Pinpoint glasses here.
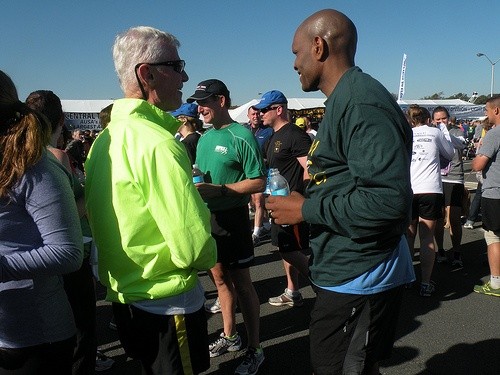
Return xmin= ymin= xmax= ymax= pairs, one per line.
xmin=260 ymin=106 xmax=278 ymax=113
xmin=135 ymin=59 xmax=187 ymax=101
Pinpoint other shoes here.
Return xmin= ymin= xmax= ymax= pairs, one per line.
xmin=464 ymin=223 xmax=474 ymax=229
xmin=251 ymin=221 xmax=273 ymax=248
xmin=109 ymin=320 xmax=117 ymax=330
xmin=436 ymin=247 xmax=464 ymax=261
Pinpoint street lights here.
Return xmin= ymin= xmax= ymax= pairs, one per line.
xmin=477 ymin=52 xmax=500 ymax=97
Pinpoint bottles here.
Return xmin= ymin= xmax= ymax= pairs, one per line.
xmin=268 ymin=168 xmax=291 ymax=227
xmin=191 ymin=164 xmax=206 ymax=184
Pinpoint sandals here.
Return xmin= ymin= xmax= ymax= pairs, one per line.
xmin=268 ymin=288 xmax=303 ymax=307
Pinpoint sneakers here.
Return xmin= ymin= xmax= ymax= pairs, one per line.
xmin=207 ymin=331 xmax=242 ymax=358
xmin=211 ymin=296 xmax=237 ymax=314
xmin=234 ymin=344 xmax=265 ymax=375
xmin=473 ymin=281 xmax=500 ymax=297
xmin=405 ymin=283 xmax=415 ymax=288
xmin=419 ymin=280 xmax=436 ymax=297
xmin=94 ymin=352 xmax=116 ymax=372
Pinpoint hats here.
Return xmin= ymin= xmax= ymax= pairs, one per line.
xmin=251 ymin=90 xmax=288 ymax=111
xmin=186 ymin=79 xmax=230 ymax=104
xmin=483 ymin=94 xmax=500 ymax=105
xmin=171 ymin=102 xmax=201 ymax=117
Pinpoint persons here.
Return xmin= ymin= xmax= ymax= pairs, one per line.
xmin=66 ymin=128 xmax=103 ymax=180
xmin=185 ymin=79 xmax=266 ymax=375
xmin=432 ymin=107 xmax=469 ymax=272
xmin=463 ymin=118 xmax=493 ymax=229
xmin=166 ymin=102 xmax=240 ymax=313
xmin=0 ymin=70 xmax=83 ymax=375
xmin=265 ymin=9 xmax=413 ymax=375
xmin=252 ymin=90 xmax=314 ymax=307
xmin=448 ymin=117 xmax=484 ymax=158
xmin=405 ymin=104 xmax=454 ymax=297
xmin=243 ymin=106 xmax=274 ymax=247
xmin=24 ymin=90 xmax=96 ymax=375
xmin=471 ymin=94 xmax=500 ymax=296
xmin=84 ymin=26 xmax=218 ymax=375
xmin=55 ymin=123 xmax=117 ymax=372
xmin=287 ymin=108 xmax=323 ymax=142
